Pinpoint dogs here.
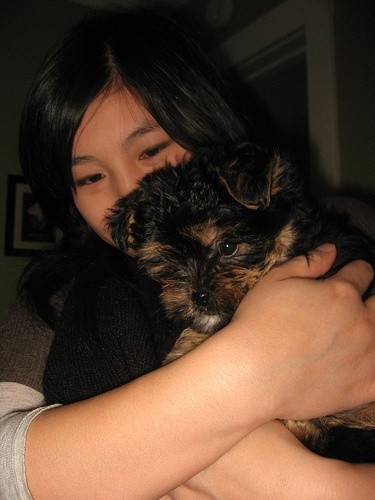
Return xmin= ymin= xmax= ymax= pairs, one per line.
xmin=101 ymin=140 xmax=373 ymax=464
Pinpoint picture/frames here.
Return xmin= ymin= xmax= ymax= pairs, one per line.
xmin=5 ymin=174 xmax=61 ymax=257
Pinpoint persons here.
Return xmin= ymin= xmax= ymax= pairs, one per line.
xmin=0 ymin=8 xmax=375 ymax=500
